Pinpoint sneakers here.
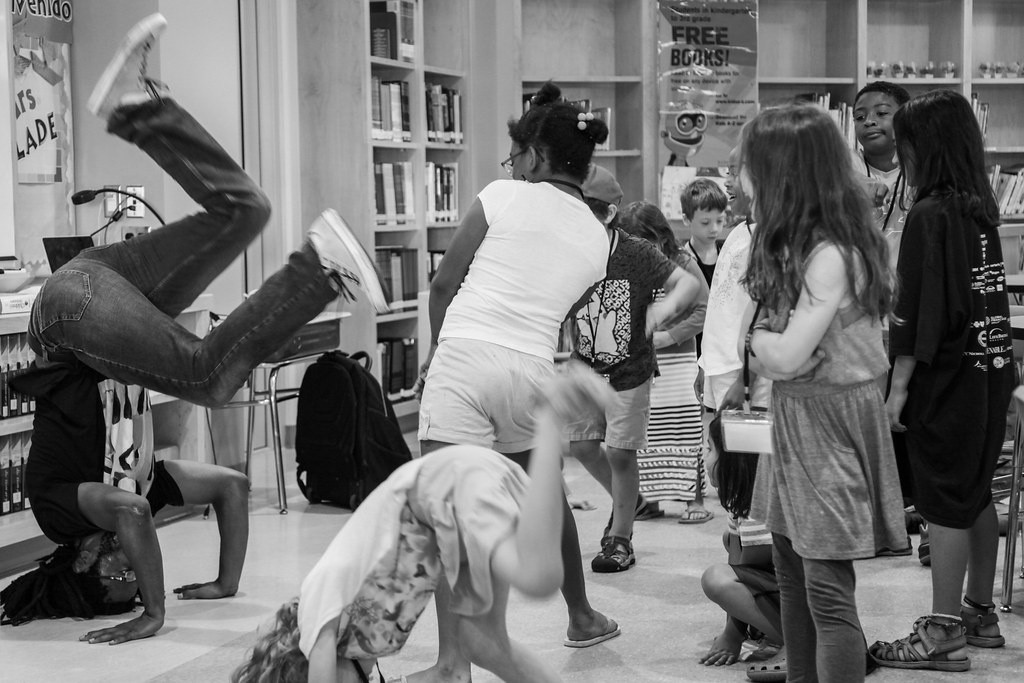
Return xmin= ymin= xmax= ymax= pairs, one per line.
xmin=309 ymin=207 xmax=391 ymax=314
xmin=87 ymin=12 xmax=167 ymax=122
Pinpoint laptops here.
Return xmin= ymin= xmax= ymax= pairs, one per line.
xmin=43 ymin=237 xmax=94 ymax=274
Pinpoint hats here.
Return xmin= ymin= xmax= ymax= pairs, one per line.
xmin=581 ymin=163 xmax=624 ymax=206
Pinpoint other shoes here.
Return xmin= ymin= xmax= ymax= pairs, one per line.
xmin=919 ymin=523 xmax=931 ymax=566
xmin=874 ymin=535 xmax=913 ymax=556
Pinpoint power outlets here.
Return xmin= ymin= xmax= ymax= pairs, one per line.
xmin=102 ymin=185 xmax=121 ymax=218
xmin=126 ymin=185 xmax=145 ymax=218
xmin=121 ymin=225 xmax=151 ymax=242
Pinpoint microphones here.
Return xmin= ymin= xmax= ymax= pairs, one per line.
xmin=71 ymin=189 xmax=166 ymax=244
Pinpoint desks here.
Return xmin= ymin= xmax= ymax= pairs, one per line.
xmin=1006 ymin=274 xmax=1024 ymax=305
xmin=1007 ymin=315 xmax=1024 ymax=339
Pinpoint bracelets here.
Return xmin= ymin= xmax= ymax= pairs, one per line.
xmin=746 ymin=327 xmax=771 ymax=357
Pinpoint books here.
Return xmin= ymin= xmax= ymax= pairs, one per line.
xmin=372 ymin=74 xmax=412 ymax=142
xmin=524 ymin=92 xmax=611 ymax=151
xmin=426 ymin=84 xmax=463 ymax=144
xmin=376 ymin=246 xmax=418 ymax=313
xmin=373 ymin=161 xmax=414 ymax=225
xmin=428 ymin=250 xmax=448 ymax=283
xmin=425 ymin=161 xmax=460 ymax=223
xmin=800 ymin=90 xmax=855 ymax=151
xmin=379 ymin=340 xmax=417 ymax=402
xmin=974 ymin=92 xmax=1024 ymax=216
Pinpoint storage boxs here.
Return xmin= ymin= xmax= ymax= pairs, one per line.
xmin=0 ymin=332 xmax=38 ymax=421
xmin=0 ymin=294 xmax=31 ymax=314
xmin=262 ymin=318 xmax=340 ymax=363
xmin=0 ymin=429 xmax=32 ymax=516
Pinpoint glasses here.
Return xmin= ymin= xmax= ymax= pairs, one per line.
xmin=503 ymin=145 xmax=544 ymax=177
xmin=98 ymin=569 xmax=137 ymax=583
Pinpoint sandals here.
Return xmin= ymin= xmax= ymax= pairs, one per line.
xmin=602 ymin=492 xmax=645 ymax=536
xmin=868 ymin=611 xmax=971 ymax=672
xmin=591 ymin=534 xmax=635 ymax=573
xmin=959 ymin=595 xmax=1006 ymax=646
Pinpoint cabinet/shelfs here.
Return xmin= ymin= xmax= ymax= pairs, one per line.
xmin=0 ymin=292 xmax=213 ymax=578
xmin=757 ymin=0 xmax=1024 ymax=221
xmin=295 ymin=0 xmax=471 ymax=443
xmin=496 ymin=0 xmax=656 ymax=208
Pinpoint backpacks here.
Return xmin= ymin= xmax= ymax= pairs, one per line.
xmin=295 ymin=350 xmax=413 ymax=512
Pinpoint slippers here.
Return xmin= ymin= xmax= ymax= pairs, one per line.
xmin=679 ymin=508 xmax=714 ymax=524
xmin=564 ymin=616 xmax=622 ymax=648
xmin=746 ymin=644 xmax=788 ymax=682
xmin=636 ymin=509 xmax=665 ymax=520
xmin=386 ymin=675 xmax=408 ymax=683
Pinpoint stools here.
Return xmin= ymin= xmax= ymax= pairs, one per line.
xmin=1001 ymin=384 xmax=1024 ymax=613
xmin=204 ymin=348 xmax=350 ymax=519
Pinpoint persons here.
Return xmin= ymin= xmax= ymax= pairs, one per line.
xmin=622 ymin=199 xmax=713 ymax=523
xmin=868 ymin=89 xmax=1006 ymax=672
xmin=739 ymin=107 xmax=909 ymax=683
xmin=702 ymin=129 xmax=786 ymax=680
xmin=569 ymin=163 xmax=698 ymax=570
xmin=232 ymin=360 xmax=628 ymax=683
xmin=385 ymin=82 xmax=610 ymax=683
xmin=678 ymin=180 xmax=727 ymax=283
xmin=1 ymin=14 xmax=392 ymax=646
xmin=853 ymin=82 xmax=931 ymax=562
